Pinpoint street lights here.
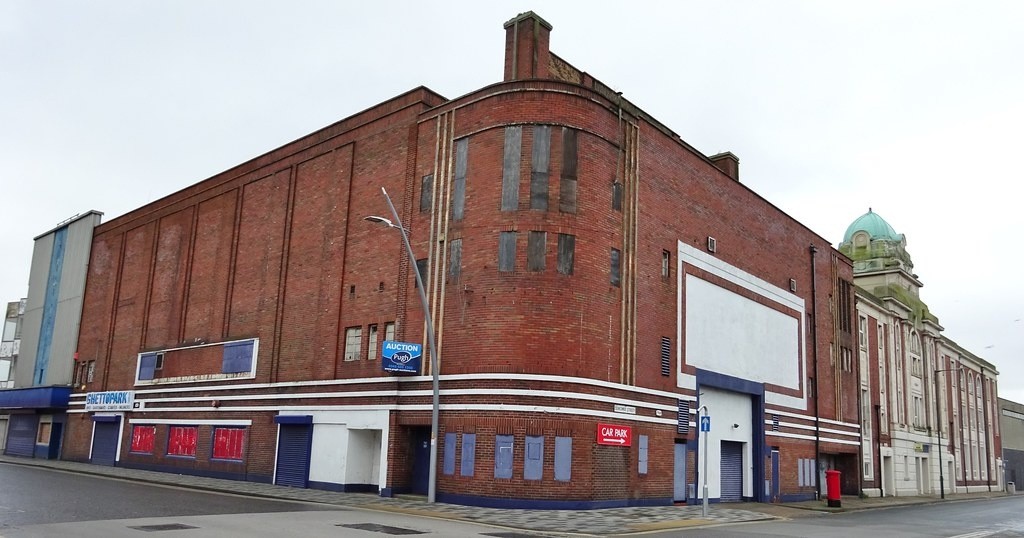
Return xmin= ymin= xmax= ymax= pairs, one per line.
xmin=363 ymin=187 xmax=440 ymax=504
xmin=934 ymin=367 xmax=962 ymax=498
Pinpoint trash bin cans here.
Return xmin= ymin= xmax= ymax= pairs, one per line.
xmin=1007 ymin=481 xmax=1015 ymax=495
